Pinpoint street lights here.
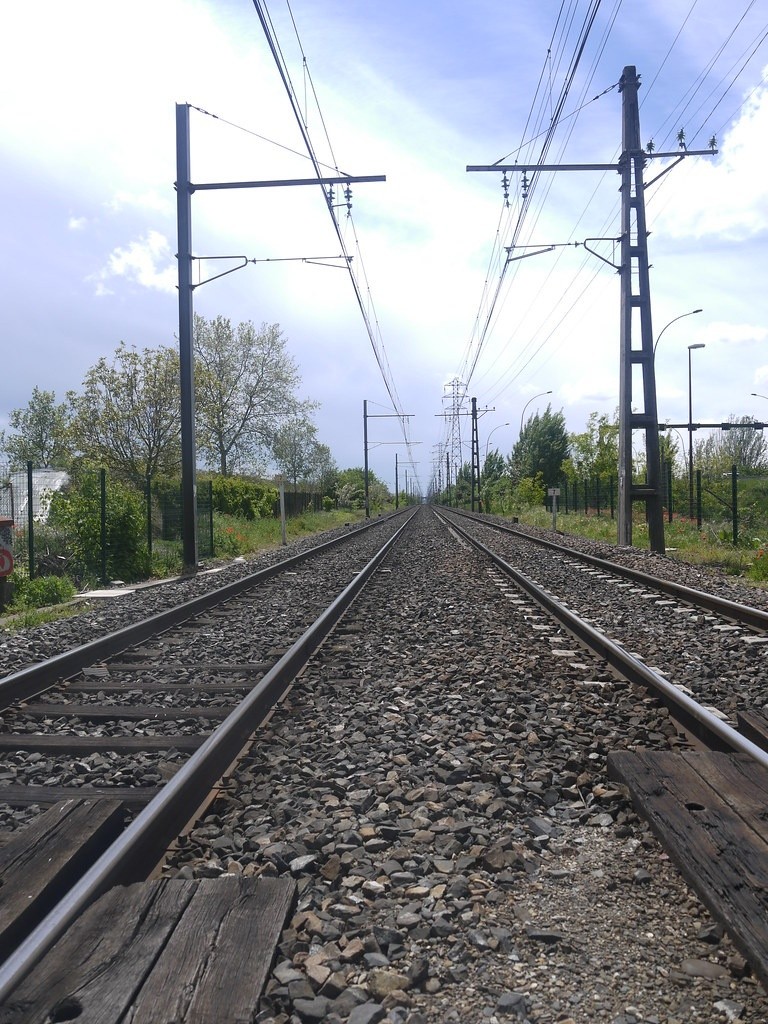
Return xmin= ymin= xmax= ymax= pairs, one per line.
xmin=521 ymin=390 xmax=553 ymax=439
xmin=687 ymin=342 xmax=707 ymax=520
xmin=486 ymin=423 xmax=510 ymax=457
